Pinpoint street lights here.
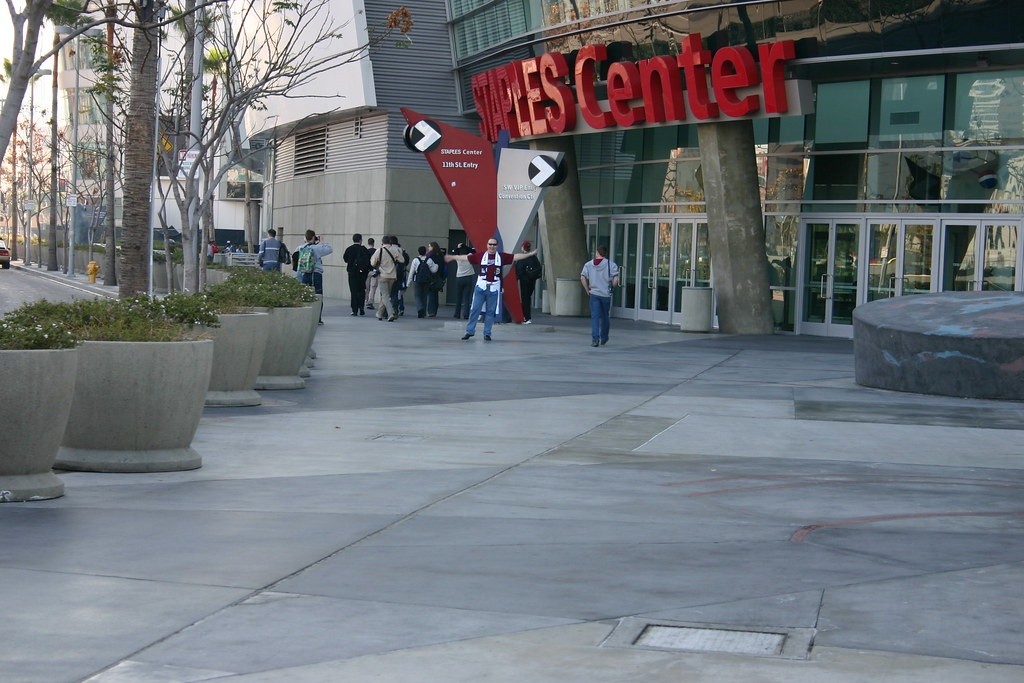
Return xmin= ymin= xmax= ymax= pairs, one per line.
xmin=24 ymin=69 xmax=53 ymax=266
xmin=52 ymin=24 xmax=102 ymax=277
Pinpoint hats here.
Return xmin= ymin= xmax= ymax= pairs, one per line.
xmin=521 ymin=241 xmax=531 ymax=249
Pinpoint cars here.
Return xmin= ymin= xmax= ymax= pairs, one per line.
xmin=0 ymin=240 xmax=11 ymax=269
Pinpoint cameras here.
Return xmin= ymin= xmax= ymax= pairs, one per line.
xmin=372 ymin=269 xmax=380 ymax=277
xmin=316 ymin=236 xmax=320 ymax=240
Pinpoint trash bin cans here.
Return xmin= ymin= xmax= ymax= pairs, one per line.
xmin=680 ymin=286 xmax=712 ymax=334
xmin=554 ymin=277 xmax=583 ymax=318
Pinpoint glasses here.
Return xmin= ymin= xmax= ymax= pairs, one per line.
xmin=442 ymin=252 xmax=445 ymax=253
xmin=488 ymin=243 xmax=497 ymax=246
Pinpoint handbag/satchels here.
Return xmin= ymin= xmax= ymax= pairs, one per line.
xmin=279 ymin=242 xmax=291 ymax=265
xmin=397 ymin=264 xmax=403 ymax=277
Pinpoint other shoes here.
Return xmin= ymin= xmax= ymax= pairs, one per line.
xmin=360 ymin=312 xmax=365 ymax=315
xmin=388 ymin=317 xmax=394 ymax=322
xmin=484 ymin=334 xmax=491 ymax=341
xmin=454 ymin=314 xmax=460 ymax=319
xmin=367 ymin=303 xmax=374 ymax=309
xmin=601 ymin=336 xmax=609 ymax=345
xmin=417 ymin=306 xmax=425 ymax=318
xmin=464 ymin=315 xmax=469 ymax=319
xmin=591 ymin=343 xmax=598 ymax=347
xmin=398 ymin=310 xmax=404 ymax=316
xmin=522 ymin=320 xmax=531 ymax=324
xmin=461 ymin=333 xmax=474 ymax=340
xmin=318 ymin=321 xmax=323 ymax=325
xmin=428 ymin=312 xmax=437 ymax=317
xmin=351 ymin=312 xmax=357 ymax=316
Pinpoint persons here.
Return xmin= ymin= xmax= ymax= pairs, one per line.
xmin=444 ymin=237 xmax=541 ymax=341
xmin=580 ymin=246 xmax=619 ymax=348
xmin=206 ymin=228 xmax=474 ymax=322
xmin=514 ymin=241 xmax=542 ymax=324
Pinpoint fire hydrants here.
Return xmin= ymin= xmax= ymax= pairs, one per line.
xmin=86 ymin=261 xmax=102 ymax=285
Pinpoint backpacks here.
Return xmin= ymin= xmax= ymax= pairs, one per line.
xmin=297 ymin=247 xmax=317 ymax=274
xmin=415 ymin=257 xmax=431 ymax=284
xmin=524 ymin=257 xmax=542 ymax=280
